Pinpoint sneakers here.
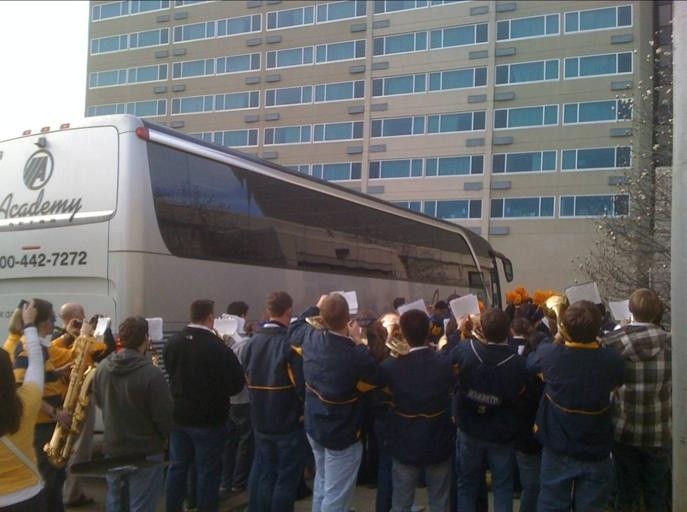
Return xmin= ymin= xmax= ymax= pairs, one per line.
xmin=410 ymin=503 xmax=425 ymax=512
xmin=220 ymin=484 xmax=245 ymax=492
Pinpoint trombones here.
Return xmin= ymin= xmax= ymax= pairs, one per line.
xmin=381 ymin=314 xmax=409 ymax=359
xmin=543 ymin=295 xmax=572 ymax=342
xmin=305 ymin=314 xmax=369 ymax=346
xmin=470 ymin=314 xmax=489 ymax=347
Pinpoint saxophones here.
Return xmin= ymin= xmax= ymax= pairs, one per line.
xmin=43 ymin=314 xmax=104 ymax=471
xmin=212 ymin=327 xmax=235 ymax=347
xmin=148 ymin=336 xmax=160 ymax=368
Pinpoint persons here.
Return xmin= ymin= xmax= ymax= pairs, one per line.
xmin=162 ymin=298 xmax=245 ymax=512
xmin=350 ymin=308 xmax=391 ymax=490
xmin=429 ymin=300 xmax=461 ymax=352
xmin=369 ymin=313 xmax=425 ymax=511
xmin=446 ymin=307 xmax=526 ymax=511
xmin=527 ymin=300 xmax=628 ymax=511
xmin=513 ymin=330 xmax=547 ymax=512
xmin=1 ymin=307 xmax=24 ymax=366
xmin=287 ymin=292 xmax=379 ymax=511
xmin=374 ymin=309 xmax=457 ymax=511
xmin=0 ymin=298 xmax=51 ymax=511
xmin=90 ymin=314 xmax=176 ymax=511
xmin=599 ymin=287 xmax=672 ymax=511
xmin=50 ymin=301 xmax=117 ymax=508
xmin=218 ymin=301 xmax=255 ymax=494
xmin=508 ymin=316 xmax=531 ymax=348
xmin=239 ymin=290 xmax=305 ymax=512
xmin=13 ymin=298 xmax=83 ymax=512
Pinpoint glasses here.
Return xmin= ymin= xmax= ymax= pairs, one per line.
xmin=45 ymin=314 xmax=57 ymax=324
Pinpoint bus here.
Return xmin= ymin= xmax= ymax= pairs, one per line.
xmin=0 ymin=115 xmax=513 ymax=459
